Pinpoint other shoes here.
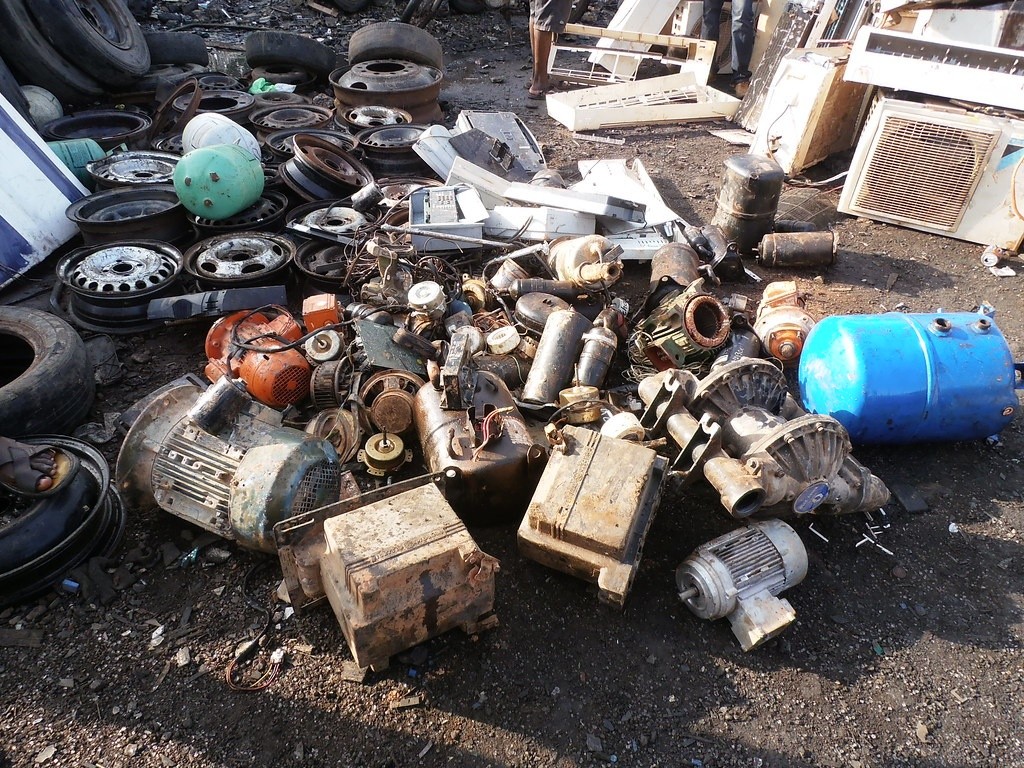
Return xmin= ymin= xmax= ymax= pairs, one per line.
xmin=735 ymin=81 xmax=749 ymax=98
xmin=707 ymin=69 xmax=717 ymax=81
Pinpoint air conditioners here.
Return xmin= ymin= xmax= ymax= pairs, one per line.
xmin=746 ymin=24 xmax=1024 ymax=253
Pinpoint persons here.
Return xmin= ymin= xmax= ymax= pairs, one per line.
xmin=0 ymin=435 xmax=80 ymax=497
xmin=528 ymin=0 xmax=573 ymax=99
xmin=702 ymin=0 xmax=754 ymax=98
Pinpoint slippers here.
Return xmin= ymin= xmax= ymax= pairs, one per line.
xmin=527 ymin=84 xmax=563 ymax=99
xmin=0 ymin=437 xmax=81 ymax=497
xmin=525 ymin=75 xmax=568 ymax=90
